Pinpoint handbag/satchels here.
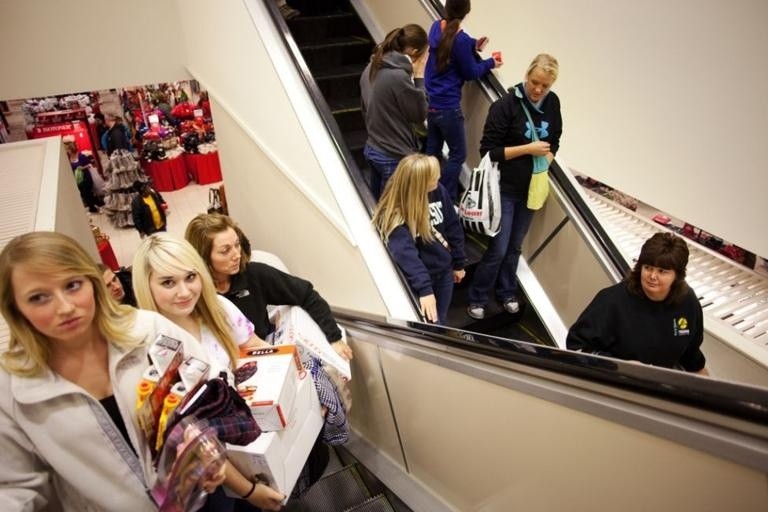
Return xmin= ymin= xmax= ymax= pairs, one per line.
xmin=208 ymin=206 xmax=223 ymax=214
xmin=526 ymin=131 xmax=549 ymax=210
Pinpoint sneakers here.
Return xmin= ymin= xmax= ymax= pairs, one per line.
xmin=503 ymin=298 xmax=519 ymax=312
xmin=467 ymin=304 xmax=485 ymax=319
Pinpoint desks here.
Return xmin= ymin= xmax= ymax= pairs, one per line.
xmin=20 ymin=85 xmax=222 ymax=192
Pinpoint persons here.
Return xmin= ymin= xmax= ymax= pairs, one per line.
xmin=370 ymin=154 xmax=466 ymax=350
xmin=359 ymin=24 xmax=430 ymax=202
xmin=566 ymin=232 xmax=709 ymax=379
xmin=466 ymin=54 xmax=562 ymax=320
xmin=423 ymin=0 xmax=503 ymax=215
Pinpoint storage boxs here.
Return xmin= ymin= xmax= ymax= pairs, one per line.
xmin=263 ymin=303 xmax=353 ymax=390
xmin=219 ymin=369 xmax=324 ymax=507
xmin=232 ymin=345 xmax=301 ymax=429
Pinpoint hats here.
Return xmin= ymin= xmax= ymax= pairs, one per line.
xmin=133 ymin=180 xmax=151 ymax=195
